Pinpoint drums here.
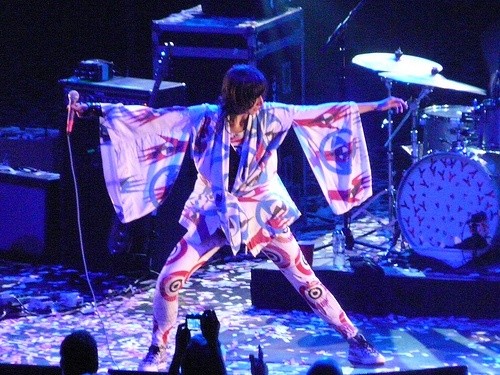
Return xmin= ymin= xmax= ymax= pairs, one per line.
xmin=394 ymin=145 xmax=500 ymax=268
xmin=422 ymin=103 xmax=483 ymax=157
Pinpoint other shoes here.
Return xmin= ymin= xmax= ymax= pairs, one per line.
xmin=138 ymin=344 xmax=168 ymax=372
xmin=347 ymin=331 xmax=385 ymax=364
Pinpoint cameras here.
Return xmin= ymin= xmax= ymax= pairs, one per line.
xmin=186 ymin=314 xmax=202 ymax=330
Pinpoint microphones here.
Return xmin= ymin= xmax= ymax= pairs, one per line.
xmin=66 ymin=89 xmax=79 ymax=133
xmin=467 ymin=211 xmax=486 ymax=224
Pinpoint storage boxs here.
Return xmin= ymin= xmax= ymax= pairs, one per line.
xmin=60 ymin=75 xmax=187 ymax=108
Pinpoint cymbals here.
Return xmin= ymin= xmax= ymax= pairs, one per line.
xmin=378 ymin=67 xmax=487 ymax=96
xmin=351 ymin=52 xmax=444 ymax=74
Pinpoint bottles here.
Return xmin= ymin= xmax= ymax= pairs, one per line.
xmin=333 ymin=225 xmax=345 ymax=268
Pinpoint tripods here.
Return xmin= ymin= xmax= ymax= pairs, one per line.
xmin=314 ymin=0 xmax=401 ymax=256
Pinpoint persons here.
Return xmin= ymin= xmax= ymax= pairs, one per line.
xmin=169 ymin=309 xmax=227 ymax=375
xmin=67 ymin=65 xmax=408 ymax=373
xmin=60 ymin=330 xmax=98 ymax=375
